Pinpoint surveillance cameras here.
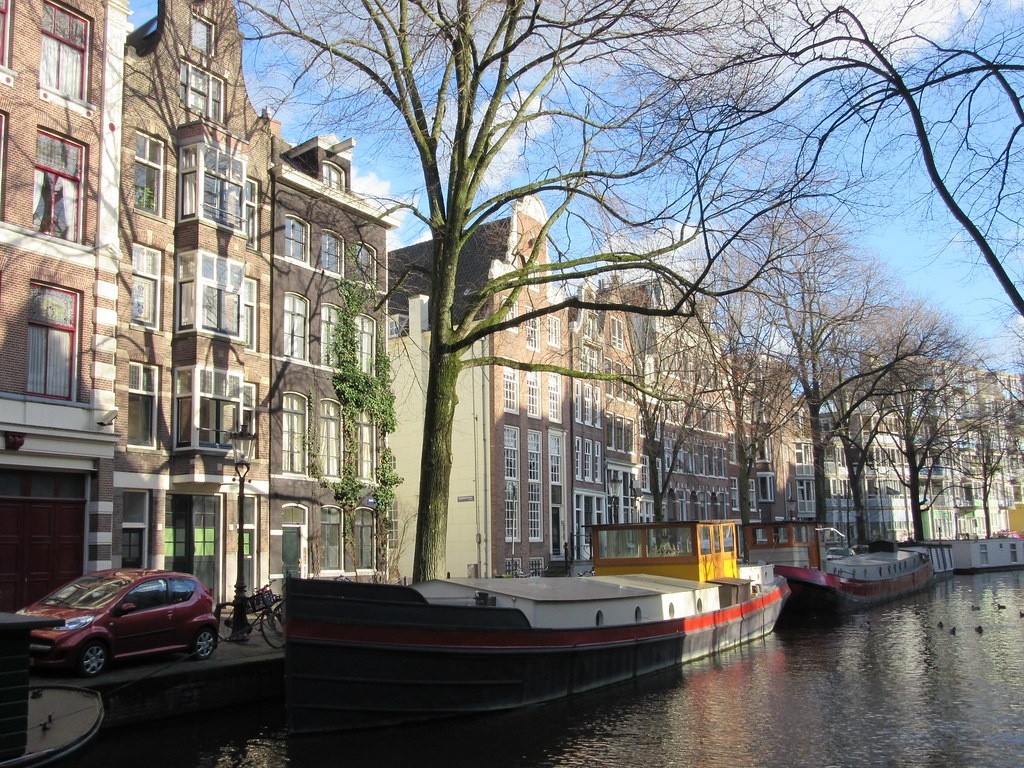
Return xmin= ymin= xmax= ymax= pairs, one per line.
xmin=97 ymin=409 xmax=117 ymax=426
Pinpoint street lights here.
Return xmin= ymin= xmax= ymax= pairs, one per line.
xmin=607 ymin=476 xmax=623 ymax=522
xmin=225 ymin=423 xmax=259 ymax=643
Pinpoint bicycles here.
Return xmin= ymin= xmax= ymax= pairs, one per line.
xmin=214 ymin=578 xmax=286 ymax=651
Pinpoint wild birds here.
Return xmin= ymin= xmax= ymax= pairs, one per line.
xmin=937 ymin=621 xmax=944 ymax=629
xmin=948 ymin=626 xmax=956 ymax=636
xmin=971 ymin=605 xmax=980 ymax=611
xmin=975 ymin=626 xmax=983 ymax=634
xmin=1019 ymin=611 xmax=1024 ymax=619
xmin=997 ymin=603 xmax=1006 ymax=610
xmin=860 ymin=621 xmax=871 ymax=630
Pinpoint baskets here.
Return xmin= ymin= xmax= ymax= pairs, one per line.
xmin=245 ymin=589 xmax=275 ymax=614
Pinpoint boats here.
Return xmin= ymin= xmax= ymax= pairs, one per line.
xmin=739 ymin=517 xmax=935 ymax=619
xmin=281 ymin=517 xmax=793 ymax=731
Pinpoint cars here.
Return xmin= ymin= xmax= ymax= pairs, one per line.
xmin=17 ymin=568 xmax=219 ymax=678
